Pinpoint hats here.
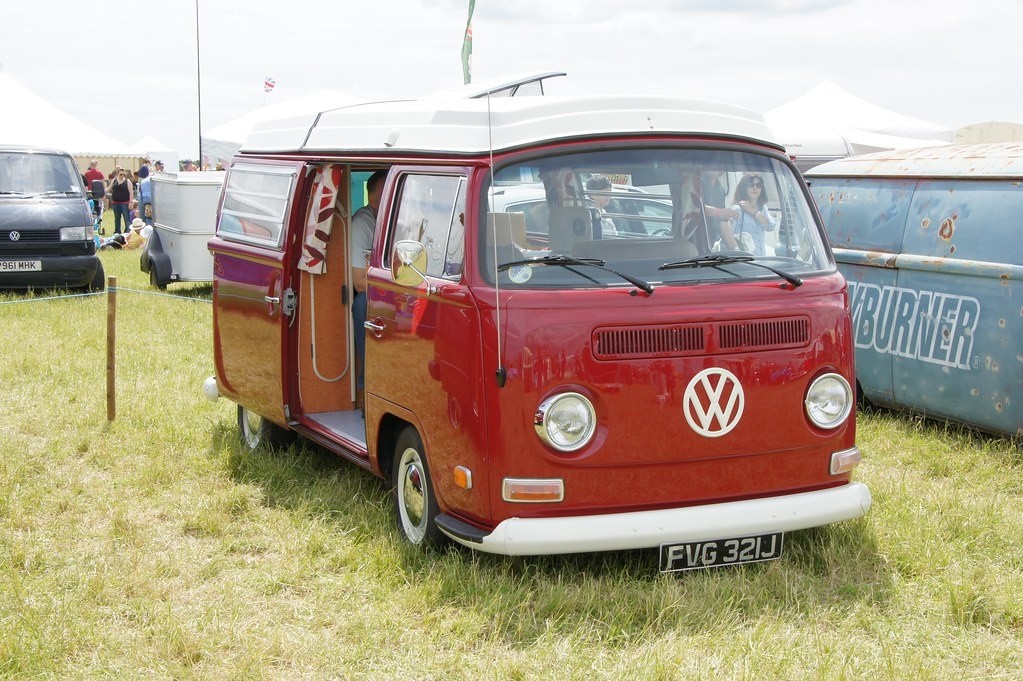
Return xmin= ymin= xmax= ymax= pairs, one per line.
xmin=129 ymin=218 xmax=145 ymax=230
xmin=138 ymin=166 xmax=149 ymax=178
xmin=181 ymin=159 xmax=193 ymax=167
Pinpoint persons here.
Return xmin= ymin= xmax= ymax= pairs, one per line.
xmin=587 ymin=175 xmax=618 ymax=239
xmin=182 ymin=159 xmax=195 ymax=172
xmin=700 ymin=171 xmax=775 ymax=257
xmin=76 ymin=159 xmax=165 ymax=250
xmin=350 ymin=170 xmax=388 ymax=387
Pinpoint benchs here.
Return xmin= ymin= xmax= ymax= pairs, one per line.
xmin=573 ymin=237 xmax=698 ymax=261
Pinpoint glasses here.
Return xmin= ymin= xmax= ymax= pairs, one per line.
xmin=747 ymin=183 xmax=761 ymax=188
xmin=120 ymin=174 xmax=125 ymax=176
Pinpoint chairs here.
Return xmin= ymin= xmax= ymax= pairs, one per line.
xmin=485 ymin=212 xmax=527 ymax=248
xmin=548 ymin=206 xmax=603 ymax=257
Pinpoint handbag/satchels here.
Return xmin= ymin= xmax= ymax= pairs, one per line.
xmin=711 ymin=232 xmax=755 ymax=254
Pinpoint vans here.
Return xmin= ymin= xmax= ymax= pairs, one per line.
xmin=350 ymin=130 xmax=1023 ymax=444
xmin=205 ymin=69 xmax=872 ymax=560
xmin=0 ymin=144 xmax=106 ymax=295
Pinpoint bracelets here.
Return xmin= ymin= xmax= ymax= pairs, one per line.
xmin=753 ymin=210 xmax=760 ymax=216
xmin=716 ymin=208 xmax=720 ymax=217
